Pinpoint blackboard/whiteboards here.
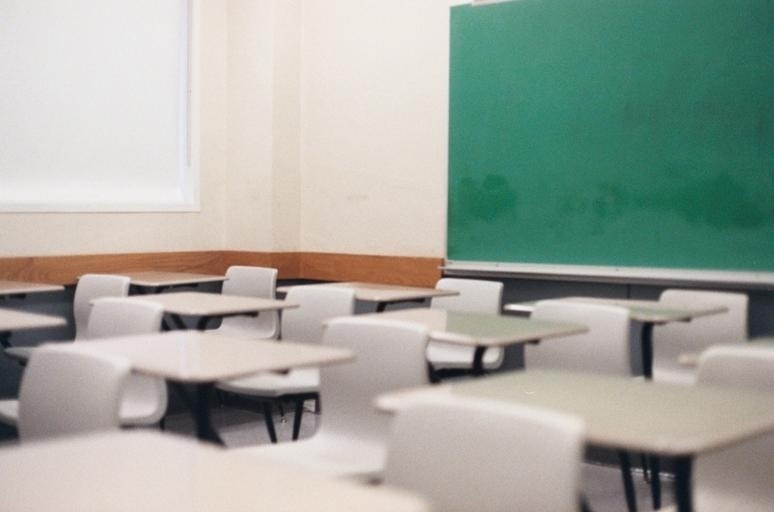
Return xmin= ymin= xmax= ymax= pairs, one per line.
xmin=438 ymin=0 xmax=774 ymax=293
